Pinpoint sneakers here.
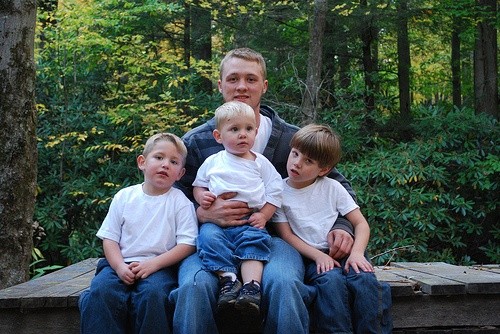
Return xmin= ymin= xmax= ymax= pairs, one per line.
xmin=237 ymin=280 xmax=262 ymax=319
xmin=216 ymin=275 xmax=242 ymax=311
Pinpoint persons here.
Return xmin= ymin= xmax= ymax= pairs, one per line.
xmin=169 ymin=47 xmax=360 ymax=334
xmin=77 ymin=131 xmax=200 ymax=334
xmin=191 ymin=100 xmax=284 ymax=320
xmin=266 ymin=122 xmax=393 ymax=334
